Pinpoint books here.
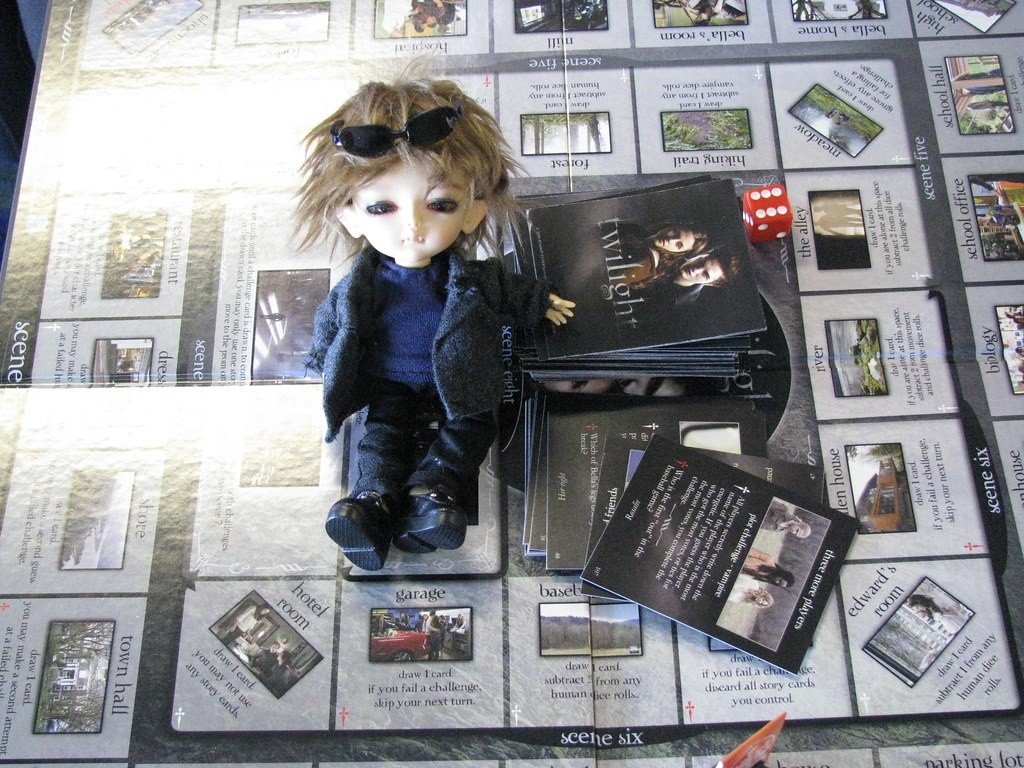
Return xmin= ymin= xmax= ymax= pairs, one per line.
xmin=498 ymin=174 xmax=860 ymax=674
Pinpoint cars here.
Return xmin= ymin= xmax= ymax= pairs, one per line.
xmin=368 ymin=626 xmax=431 ymax=662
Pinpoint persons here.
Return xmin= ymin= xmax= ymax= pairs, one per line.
xmin=908 ymin=596 xmax=967 ymax=633
xmin=999 ymin=306 xmax=1024 ymax=390
xmin=740 ymin=547 xmax=795 ymax=588
xmin=728 ymin=574 xmax=773 ymax=608
xmin=616 ymin=377 xmax=685 ymax=397
xmin=371 ymin=610 xmax=466 ymax=660
xmin=542 ymin=378 xmax=613 ymax=394
xmin=828 ymin=109 xmax=849 ymax=125
xmin=223 ymin=603 xmax=292 ymax=699
xmin=760 ymin=499 xmax=811 ymax=539
xmin=289 ymin=58 xmax=576 ymax=571
xmin=604 ymin=221 xmax=708 ymax=290
xmin=382 ymin=0 xmax=456 ymax=38
xmin=612 ymin=246 xmax=741 ymax=327
xmin=969 ymin=175 xmax=1024 ymax=260
